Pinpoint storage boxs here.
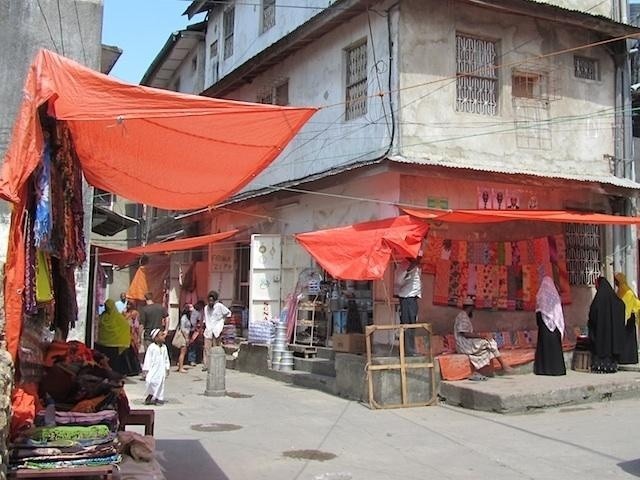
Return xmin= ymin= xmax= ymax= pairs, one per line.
xmin=333 ymin=332 xmax=366 ymax=353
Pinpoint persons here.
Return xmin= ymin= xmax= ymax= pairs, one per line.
xmin=493 ymin=191 xmax=506 ymax=210
xmin=532 ymin=275 xmax=566 ymax=376
xmin=528 ymin=196 xmax=536 ymax=209
xmin=453 ymin=298 xmax=519 ymax=375
xmin=95 ymin=289 xmax=233 ymax=372
xmin=614 ymin=272 xmax=640 ymax=365
xmin=507 ymin=196 xmax=519 ymax=209
xmin=142 ymin=326 xmax=171 ymax=405
xmin=587 ymin=277 xmax=625 ymax=373
xmin=479 ymin=190 xmax=491 ymax=210
xmin=393 ymin=250 xmax=423 ymax=357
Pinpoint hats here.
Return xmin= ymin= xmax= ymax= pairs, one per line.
xmin=150 ymin=329 xmax=160 ymax=341
xmin=462 ymin=298 xmax=474 ymax=306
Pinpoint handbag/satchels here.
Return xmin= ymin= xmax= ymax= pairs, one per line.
xmin=171 ymin=327 xmax=184 ymax=349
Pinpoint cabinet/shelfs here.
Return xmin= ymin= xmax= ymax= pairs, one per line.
xmin=293 ymin=293 xmax=332 ymax=348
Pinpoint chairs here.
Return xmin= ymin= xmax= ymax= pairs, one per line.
xmin=117 ymin=387 xmax=155 ymax=436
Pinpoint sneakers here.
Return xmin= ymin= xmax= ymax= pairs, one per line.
xmin=400 ymin=350 xmax=422 ymax=358
xmin=175 ymin=362 xmax=206 ymax=374
xmin=144 ymin=395 xmax=164 ymax=406
